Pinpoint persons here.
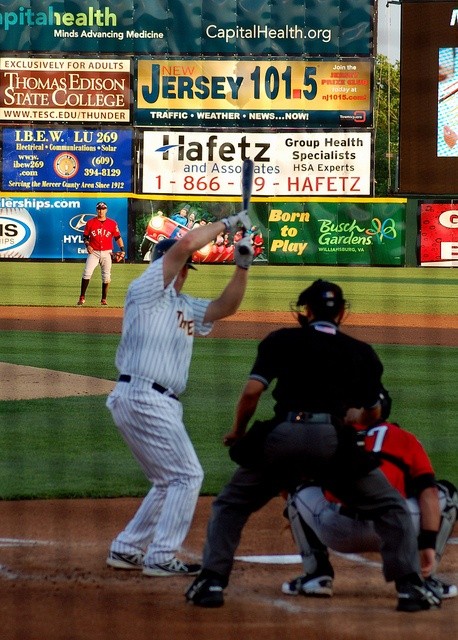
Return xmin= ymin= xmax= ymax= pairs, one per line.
xmin=169 ymin=208 xmax=187 ymax=226
xmin=255 ymin=232 xmax=265 ymax=248
xmin=217 ymin=233 xmax=227 ymax=244
xmin=188 ymin=213 xmax=196 ymax=226
xmin=247 ymin=224 xmax=258 ymax=236
xmin=182 ymin=276 xmax=446 ymax=613
xmin=77 ymin=202 xmax=126 ymax=311
xmin=224 ymin=234 xmax=230 ymax=246
xmin=233 ymin=231 xmax=246 ymax=244
xmin=281 ymin=386 xmax=458 ymax=600
xmin=199 ymin=219 xmax=206 ymax=225
xmin=103 ymin=209 xmax=261 ymax=579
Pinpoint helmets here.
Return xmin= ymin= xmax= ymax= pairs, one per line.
xmin=290 ymin=279 xmax=351 ymax=326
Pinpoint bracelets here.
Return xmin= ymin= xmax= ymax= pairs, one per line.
xmin=420 ymin=527 xmax=439 ymax=549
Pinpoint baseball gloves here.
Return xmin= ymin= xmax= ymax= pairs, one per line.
xmin=111 ymin=251 xmax=127 ymax=263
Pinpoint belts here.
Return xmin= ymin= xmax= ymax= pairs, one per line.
xmin=285 ymin=412 xmax=331 ymax=424
xmin=119 ymin=375 xmax=179 ymax=401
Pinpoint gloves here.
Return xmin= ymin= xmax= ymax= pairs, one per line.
xmin=234 ymin=237 xmax=253 ymax=270
xmin=220 ymin=210 xmax=251 ymax=231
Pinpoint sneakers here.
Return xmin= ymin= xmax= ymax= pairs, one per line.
xmin=423 ymin=576 xmax=458 ymax=598
xmin=282 ymin=576 xmax=333 ymax=598
xmin=102 ymin=299 xmax=106 ymax=304
xmin=397 ymin=584 xmax=441 ymax=612
xmin=185 ymin=579 xmax=223 ymax=608
xmin=142 ymin=557 xmax=202 ymax=577
xmin=106 ymin=550 xmax=144 ymax=569
xmin=78 ymin=296 xmax=85 ymax=304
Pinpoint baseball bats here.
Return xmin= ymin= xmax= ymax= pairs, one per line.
xmin=239 ymin=160 xmax=253 ymax=255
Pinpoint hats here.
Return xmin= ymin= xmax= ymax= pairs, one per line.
xmin=152 ymin=239 xmax=198 ymax=271
xmin=96 ymin=202 xmax=107 ymax=210
